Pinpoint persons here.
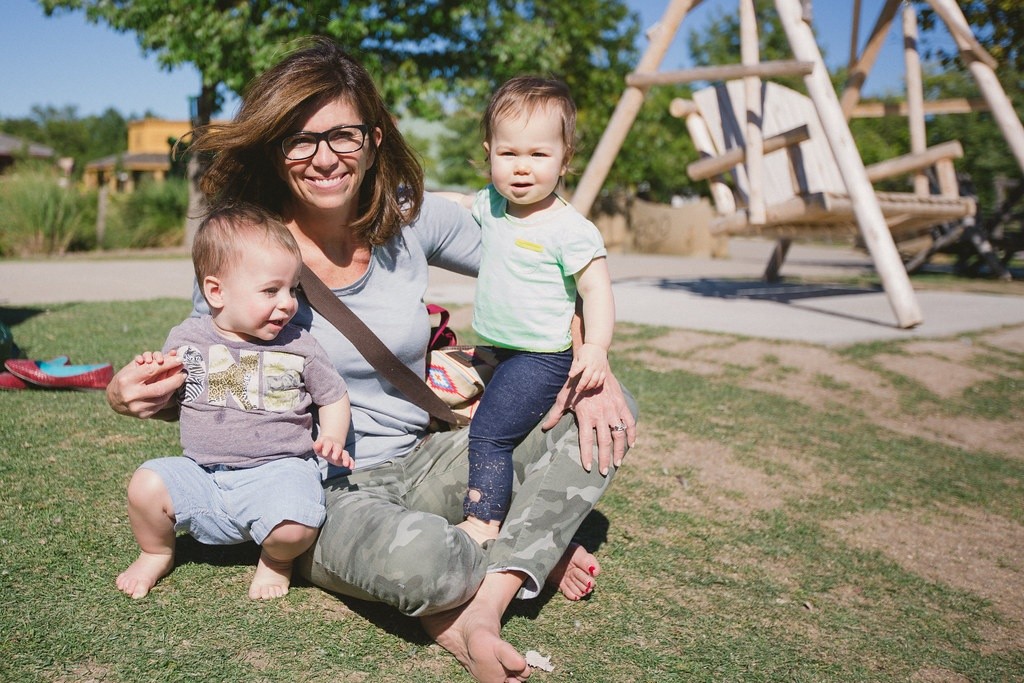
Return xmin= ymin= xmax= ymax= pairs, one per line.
xmin=106 ymin=35 xmax=642 ymax=683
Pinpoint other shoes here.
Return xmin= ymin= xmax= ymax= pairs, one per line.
xmin=4 ymin=359 xmax=115 ymax=389
xmin=1 ymin=355 xmax=72 ymax=390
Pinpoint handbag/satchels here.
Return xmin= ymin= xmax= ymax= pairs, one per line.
xmin=426 ymin=304 xmax=457 ymax=353
xmin=425 ymin=345 xmax=517 ymax=427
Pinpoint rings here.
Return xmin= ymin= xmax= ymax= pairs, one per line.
xmin=610 ymin=422 xmax=627 ymax=432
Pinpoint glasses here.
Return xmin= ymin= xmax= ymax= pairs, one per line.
xmin=276 ymin=125 xmax=369 ymax=160
